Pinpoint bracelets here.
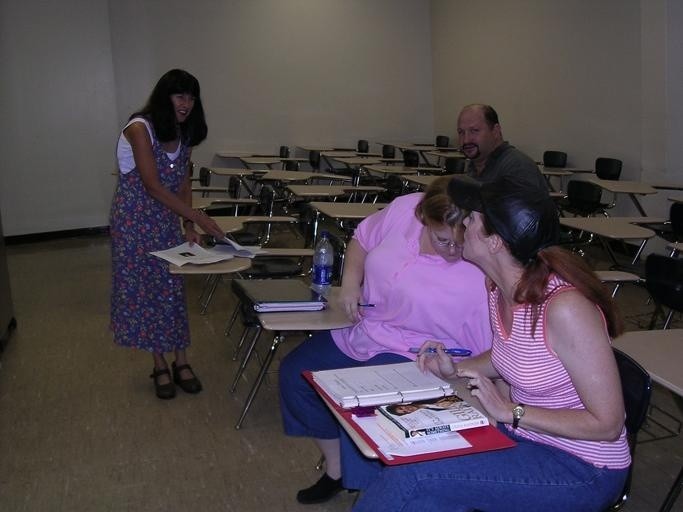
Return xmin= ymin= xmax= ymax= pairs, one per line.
xmin=182 ymin=218 xmax=193 ymax=228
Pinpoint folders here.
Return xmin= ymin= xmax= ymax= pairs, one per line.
xmin=300 ymin=360 xmax=519 ymax=467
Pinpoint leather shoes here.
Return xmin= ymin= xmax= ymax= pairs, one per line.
xmin=296 ymin=472 xmax=360 ymax=505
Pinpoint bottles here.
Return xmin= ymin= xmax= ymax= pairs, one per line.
xmin=311 ymin=231 xmax=334 ymax=299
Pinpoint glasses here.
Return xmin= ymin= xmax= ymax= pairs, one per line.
xmin=428 ymin=220 xmax=464 ymax=248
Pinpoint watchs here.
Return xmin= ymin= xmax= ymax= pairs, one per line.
xmin=511 ymin=403 xmax=526 ymax=430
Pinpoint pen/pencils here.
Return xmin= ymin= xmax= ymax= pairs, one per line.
xmin=409 ymin=347 xmax=472 ymax=356
xmin=358 ymin=303 xmax=377 ymax=307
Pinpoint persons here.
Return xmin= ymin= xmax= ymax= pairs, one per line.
xmin=457 ymin=103 xmax=551 ymax=188
xmin=350 ymin=176 xmax=634 ymax=512
xmin=108 ymin=68 xmax=226 ymax=400
xmin=409 ymin=428 xmax=426 ymax=438
xmin=385 ymin=396 xmax=464 ymax=416
xmin=277 ymin=175 xmax=500 ymax=504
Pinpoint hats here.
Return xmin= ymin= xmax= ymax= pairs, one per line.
xmin=446 ymin=174 xmax=562 ymax=263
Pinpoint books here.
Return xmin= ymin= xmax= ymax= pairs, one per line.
xmin=373 ymin=394 xmax=492 ymax=440
xmin=230 ymin=277 xmax=328 ymax=313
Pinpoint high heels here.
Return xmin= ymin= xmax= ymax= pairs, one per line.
xmin=171 ymin=360 xmax=201 ymax=393
xmin=149 ymin=366 xmax=175 ymax=400
